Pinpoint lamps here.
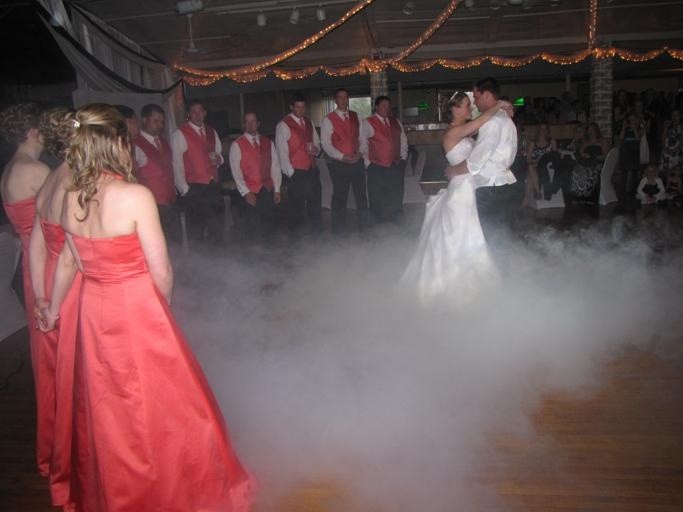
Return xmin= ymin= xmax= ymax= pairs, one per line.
xmin=288 ymin=6 xmax=300 ymax=26
xmin=403 ymin=1 xmax=416 ymax=16
xmin=255 ymin=8 xmax=268 ymax=28
xmin=315 ymin=4 xmax=327 ymax=21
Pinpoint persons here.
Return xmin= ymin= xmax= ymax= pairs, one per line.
xmin=445 ymin=77 xmax=519 ymax=270
xmin=501 ymin=91 xmax=682 ymax=229
xmin=130 ymin=104 xmax=177 ymax=228
xmin=228 ymin=88 xmax=408 ymax=236
xmin=1 ymin=104 xmax=80 ymax=512
xmin=169 ymin=98 xmax=225 ymax=254
xmin=401 ymin=91 xmax=514 ymax=300
xmin=60 ymin=103 xmax=260 ymax=512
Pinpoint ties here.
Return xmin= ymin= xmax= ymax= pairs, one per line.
xmin=384 ymin=118 xmax=388 ymax=128
xmin=253 ymin=138 xmax=258 ymax=151
xmin=300 ymin=119 xmax=304 ymax=128
xmin=199 ymin=128 xmax=204 ymax=136
xmin=343 ymin=113 xmax=348 ymax=120
xmin=155 ymin=138 xmax=160 ymax=149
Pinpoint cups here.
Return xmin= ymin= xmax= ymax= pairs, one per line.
xmin=304 ymin=142 xmax=313 ymax=155
xmin=209 ymin=152 xmax=218 ymax=165
